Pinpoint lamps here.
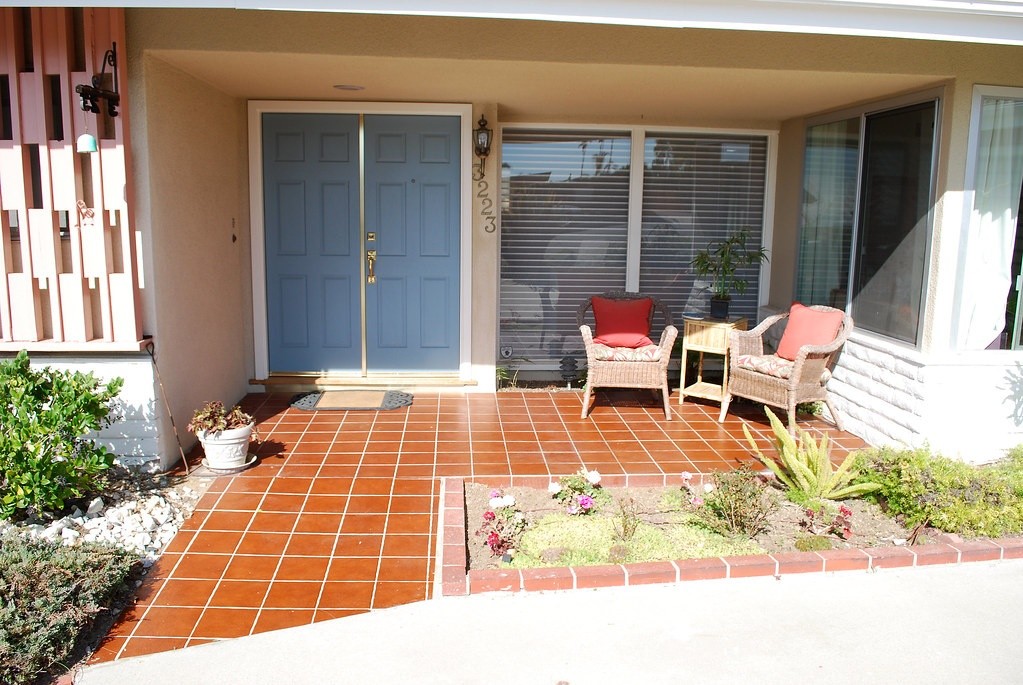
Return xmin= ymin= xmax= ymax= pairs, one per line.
xmin=472 ymin=114 xmax=493 ymax=178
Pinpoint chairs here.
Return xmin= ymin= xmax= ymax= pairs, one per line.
xmin=719 ymin=305 xmax=854 ymax=435
xmin=576 ymin=292 xmax=678 ymax=421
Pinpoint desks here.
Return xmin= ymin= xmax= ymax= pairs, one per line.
xmin=679 ymin=316 xmax=749 ymax=406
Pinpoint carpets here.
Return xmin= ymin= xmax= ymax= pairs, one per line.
xmin=289 ymin=390 xmax=413 ymax=410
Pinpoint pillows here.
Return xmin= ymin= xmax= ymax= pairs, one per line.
xmin=592 ymin=297 xmax=652 ymax=347
xmin=774 ymin=301 xmax=843 ymax=361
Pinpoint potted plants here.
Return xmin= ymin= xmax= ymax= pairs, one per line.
xmin=690 ymin=228 xmax=771 ymax=319
xmin=186 ymin=400 xmax=262 ymax=475
